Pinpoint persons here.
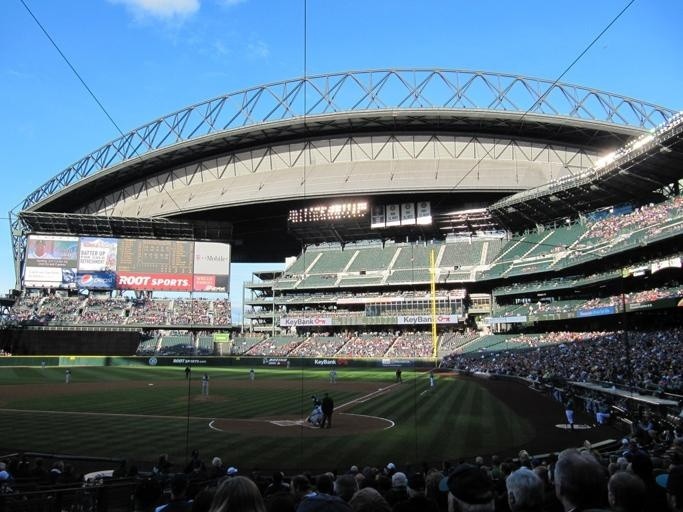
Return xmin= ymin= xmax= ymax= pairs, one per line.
xmin=1 ymin=194 xmax=682 ymax=511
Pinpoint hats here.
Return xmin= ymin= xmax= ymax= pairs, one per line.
xmin=391 ymin=471 xmax=408 ymax=488
xmin=386 ymin=462 xmax=396 ymax=470
xmin=438 ymin=463 xmax=493 ymax=504
xmin=226 ymin=466 xmax=238 ymax=474
xmin=655 ymin=467 xmax=683 ymax=490
xmin=350 ymin=465 xmax=358 ymax=472
